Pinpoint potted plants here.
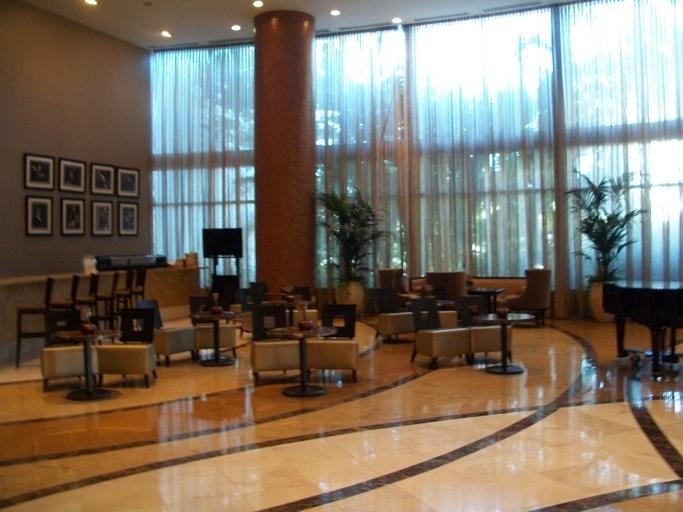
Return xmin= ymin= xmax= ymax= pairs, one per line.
xmin=315 ymin=187 xmax=389 ymax=317
xmin=563 ymin=172 xmax=648 ymax=323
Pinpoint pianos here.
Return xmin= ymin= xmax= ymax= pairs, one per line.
xmin=602 ymin=281 xmax=683 ymax=375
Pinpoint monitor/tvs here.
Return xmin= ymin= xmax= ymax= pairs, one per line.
xmin=202 ymin=228 xmax=242 ymax=257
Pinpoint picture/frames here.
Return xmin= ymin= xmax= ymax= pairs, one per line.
xmin=118 ymin=202 xmax=139 ymax=236
xmin=24 ymin=153 xmax=54 ymax=190
xmin=91 ymin=163 xmax=115 ymax=196
xmin=61 ymin=197 xmax=85 ymax=236
xmin=117 ymin=168 xmax=139 ymax=198
xmin=92 ymin=200 xmax=114 ymax=236
xmin=26 ymin=195 xmax=52 ymax=236
xmin=59 ymin=158 xmax=86 ymax=193
